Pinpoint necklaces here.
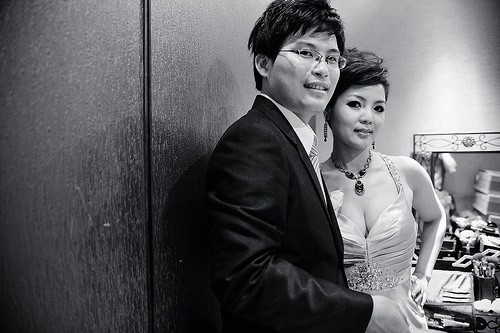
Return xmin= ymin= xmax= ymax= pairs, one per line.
xmin=330 ymin=149 xmax=373 ymax=195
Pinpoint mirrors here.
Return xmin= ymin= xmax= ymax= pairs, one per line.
xmin=413 ymin=132 xmax=500 ymax=267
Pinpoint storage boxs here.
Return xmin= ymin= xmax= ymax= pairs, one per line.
xmin=471 ymin=168 xmax=500 ymax=233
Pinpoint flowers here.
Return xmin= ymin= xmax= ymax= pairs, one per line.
xmin=328 ymin=190 xmax=344 ymax=217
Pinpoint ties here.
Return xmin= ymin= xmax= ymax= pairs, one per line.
xmin=309 ymin=143 xmax=328 ymax=208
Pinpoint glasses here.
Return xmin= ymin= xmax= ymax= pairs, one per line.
xmin=277 ymin=48 xmax=347 ymax=70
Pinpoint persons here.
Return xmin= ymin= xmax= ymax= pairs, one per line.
xmin=315 ymin=46 xmax=447 ymax=333
xmin=201 ymin=0 xmax=411 ymax=333
